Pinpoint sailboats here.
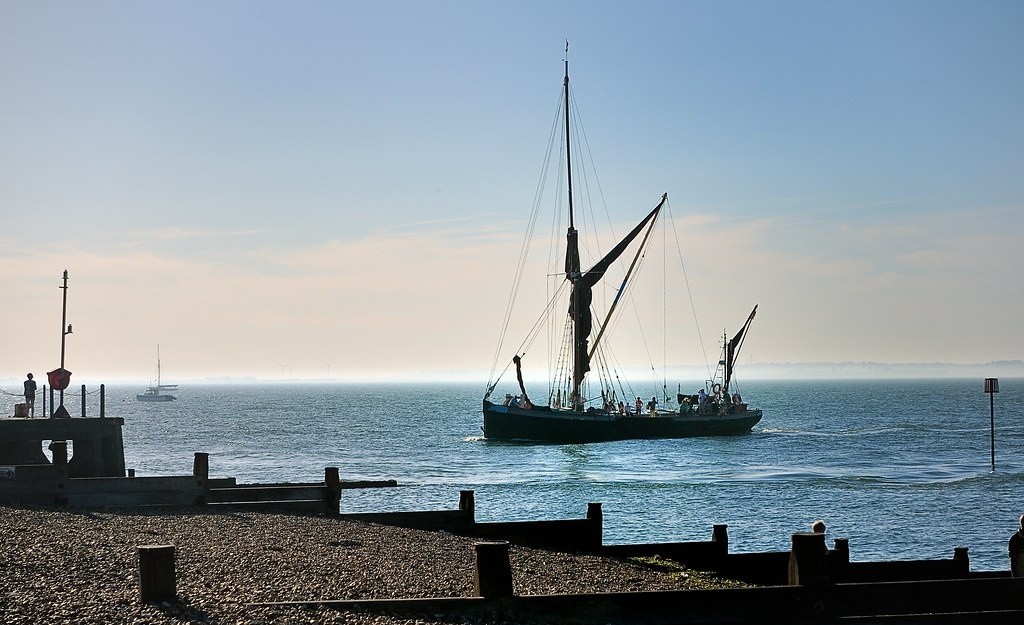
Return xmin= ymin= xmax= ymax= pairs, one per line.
xmin=149 ymin=343 xmax=178 ymax=391
xmin=483 ymin=41 xmax=764 ymax=442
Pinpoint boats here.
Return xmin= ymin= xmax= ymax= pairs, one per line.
xmin=136 ymin=387 xmax=177 ymax=401
xmin=0 ymin=269 xmax=342 ymax=516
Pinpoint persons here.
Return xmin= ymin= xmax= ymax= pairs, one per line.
xmin=608 ymin=396 xmax=658 ymax=417
xmin=510 ymin=396 xmax=521 ymax=408
xmin=24 ymin=373 xmax=37 ymax=418
xmin=679 ymin=389 xmax=731 ymax=416
xmin=573 ymin=394 xmax=587 ymax=412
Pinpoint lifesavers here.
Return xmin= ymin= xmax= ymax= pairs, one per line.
xmin=713 ymin=383 xmax=722 ymax=394
